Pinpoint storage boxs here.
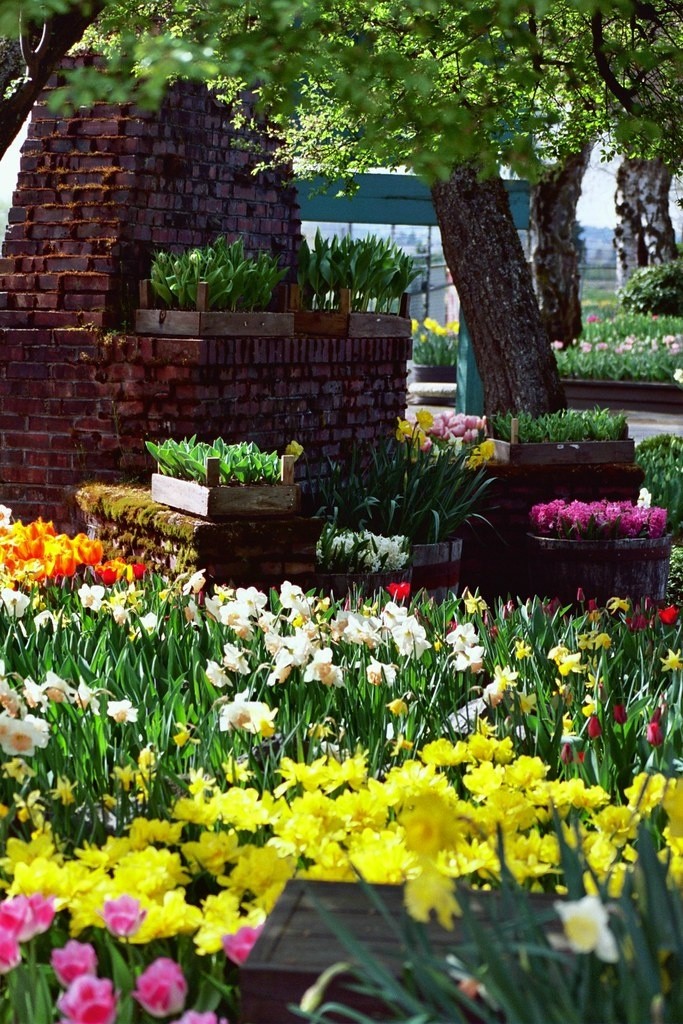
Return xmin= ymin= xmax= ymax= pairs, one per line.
xmin=234 ymin=878 xmax=568 ymax=1024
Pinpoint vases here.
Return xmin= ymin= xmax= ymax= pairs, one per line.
xmin=527 ymin=532 xmax=673 ymax=603
xmin=315 ymin=571 xmax=411 ymax=605
xmin=413 ymin=539 xmax=464 ymax=603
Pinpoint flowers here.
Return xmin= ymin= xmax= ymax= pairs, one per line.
xmin=0 ymin=413 xmax=683 ymax=1024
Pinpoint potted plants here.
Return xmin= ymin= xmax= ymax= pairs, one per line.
xmin=289 ymin=225 xmax=422 ymax=339
xmin=134 ymin=235 xmax=297 ymax=337
xmin=147 ymin=437 xmax=297 ymax=516
xmin=489 ymin=407 xmax=635 ymax=465
